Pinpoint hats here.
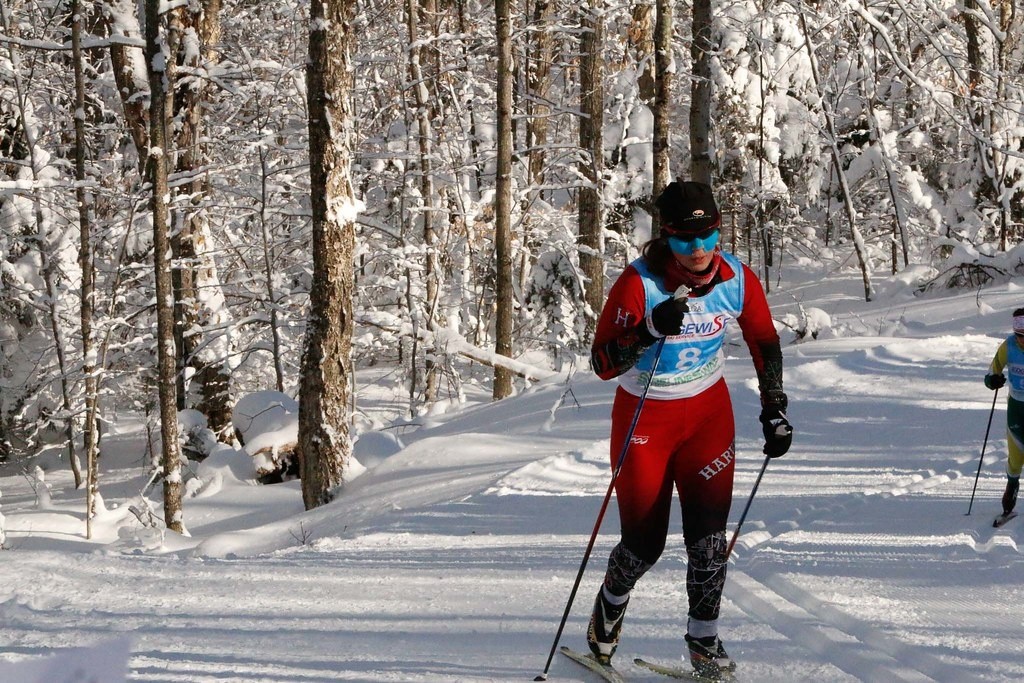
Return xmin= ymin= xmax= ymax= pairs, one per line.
xmin=656 ymin=180 xmax=719 ymax=229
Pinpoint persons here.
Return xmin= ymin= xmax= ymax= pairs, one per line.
xmin=984 ymin=307 xmax=1024 ymax=528
xmin=586 ymin=182 xmax=793 ymax=683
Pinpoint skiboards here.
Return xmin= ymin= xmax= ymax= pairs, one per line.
xmin=556 ymin=645 xmax=721 ymax=683
xmin=993 ymin=513 xmax=1019 ymax=528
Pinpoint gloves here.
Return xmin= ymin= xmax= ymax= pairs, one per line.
xmin=634 ymin=296 xmax=689 ymax=347
xmin=987 ymin=373 xmax=1007 ymax=390
xmin=760 ymin=403 xmax=792 ymax=458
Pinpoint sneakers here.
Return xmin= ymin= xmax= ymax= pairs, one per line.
xmin=1002 ymin=480 xmax=1019 ymax=512
xmin=587 ymin=583 xmax=630 ymax=664
xmin=683 ymin=632 xmax=736 ymax=679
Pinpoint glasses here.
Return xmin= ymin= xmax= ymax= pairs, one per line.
xmin=1014 ymin=331 xmax=1024 ymax=338
xmin=674 ymin=226 xmax=716 ymax=242
